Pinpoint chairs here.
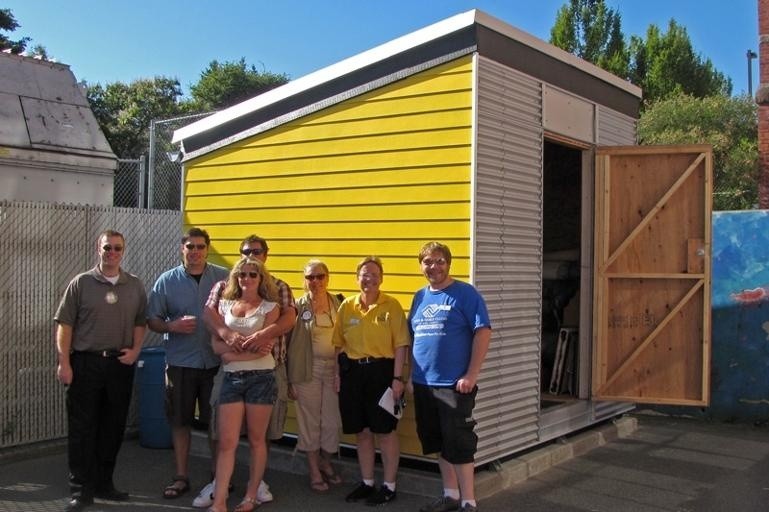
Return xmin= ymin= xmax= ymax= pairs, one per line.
xmin=364 ymin=485 xmax=396 ymax=506
xmin=193 ymin=478 xmax=229 ymax=508
xmin=345 ymin=484 xmax=377 ymax=502
xmin=248 ymin=479 xmax=273 ymax=503
xmin=421 ymin=494 xmax=480 ymax=512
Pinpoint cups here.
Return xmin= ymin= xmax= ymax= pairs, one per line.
xmin=239 ymin=272 xmax=257 ymax=278
xmin=306 ymin=274 xmax=325 ymax=280
xmin=103 ymin=245 xmax=122 ymax=252
xmin=242 ymin=248 xmax=265 ymax=255
xmin=186 ymin=244 xmax=205 ymax=250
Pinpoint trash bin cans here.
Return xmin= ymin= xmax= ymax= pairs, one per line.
xmin=393 ymin=376 xmax=404 ymax=383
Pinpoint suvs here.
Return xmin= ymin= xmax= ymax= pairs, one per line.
xmin=134 ymin=346 xmax=175 ymax=450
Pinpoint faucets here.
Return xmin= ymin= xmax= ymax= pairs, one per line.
xmin=164 ymin=475 xmax=188 ymax=499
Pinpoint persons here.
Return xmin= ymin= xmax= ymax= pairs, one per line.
xmin=205 ymin=256 xmax=281 ymax=512
xmin=189 ymin=235 xmax=299 ymax=511
xmin=52 ymin=230 xmax=148 ymax=510
xmin=287 ymin=260 xmax=345 ymax=494
xmin=331 ymin=255 xmax=412 ymax=506
xmin=146 ymin=229 xmax=233 ymax=498
xmin=407 ymin=241 xmax=491 ymax=512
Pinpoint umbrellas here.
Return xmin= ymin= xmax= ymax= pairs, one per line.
xmin=94 ymin=489 xmax=129 ymax=500
xmin=64 ymin=498 xmax=93 ymax=511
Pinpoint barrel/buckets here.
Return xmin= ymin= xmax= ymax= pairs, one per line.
xmin=233 ymin=497 xmax=262 ymax=512
xmin=320 ymin=465 xmax=341 ymax=484
xmin=309 ymin=471 xmax=329 ymax=493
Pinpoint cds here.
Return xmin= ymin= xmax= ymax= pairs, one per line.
xmin=394 ymin=396 xmax=407 ymax=416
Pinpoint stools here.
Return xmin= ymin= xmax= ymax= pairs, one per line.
xmin=97 ymin=350 xmax=120 ymax=358
xmin=352 ymin=357 xmax=385 ymax=364
